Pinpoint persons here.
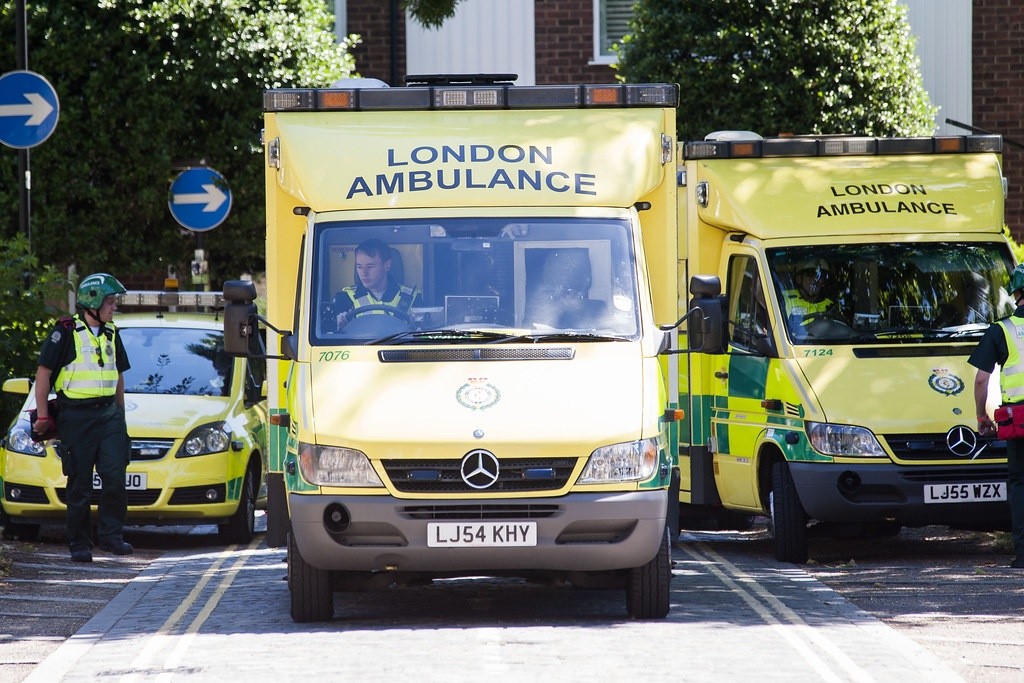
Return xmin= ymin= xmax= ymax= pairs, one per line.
xmin=967 ymin=265 xmax=1024 ymax=569
xmin=333 ymin=239 xmax=424 ymax=330
xmin=755 ymin=256 xmax=840 ymax=336
xmin=33 ymin=273 xmax=131 ymax=561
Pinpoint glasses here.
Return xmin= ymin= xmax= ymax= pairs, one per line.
xmin=802 ymin=270 xmax=826 ymax=277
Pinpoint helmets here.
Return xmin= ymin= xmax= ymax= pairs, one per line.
xmin=793 ymin=254 xmax=830 ymax=274
xmin=1007 ymin=263 xmax=1024 ymax=296
xmin=76 ymin=272 xmax=128 ymax=310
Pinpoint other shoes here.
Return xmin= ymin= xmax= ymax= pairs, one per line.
xmin=1010 ymin=555 xmax=1023 ymax=568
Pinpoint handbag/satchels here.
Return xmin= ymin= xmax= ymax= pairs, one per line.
xmin=993 ymin=404 xmax=1024 ymax=441
xmin=29 ymin=398 xmax=58 ymax=444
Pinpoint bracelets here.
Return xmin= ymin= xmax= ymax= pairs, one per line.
xmin=977 ymin=414 xmax=989 ymax=423
xmin=37 ymin=416 xmax=49 ymax=420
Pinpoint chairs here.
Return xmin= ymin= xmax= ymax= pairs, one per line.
xmin=353 ymin=249 xmax=404 ymax=286
xmin=527 ymin=248 xmax=610 ymax=329
xmin=885 ymin=278 xmax=957 ymax=327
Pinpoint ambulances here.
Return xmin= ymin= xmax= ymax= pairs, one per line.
xmin=259 ymin=74 xmax=681 ymax=621
xmin=675 ymin=129 xmax=1021 ymax=562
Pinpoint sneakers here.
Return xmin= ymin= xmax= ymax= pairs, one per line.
xmin=99 ymin=536 xmax=135 ymax=556
xmin=71 ymin=547 xmax=93 ymax=562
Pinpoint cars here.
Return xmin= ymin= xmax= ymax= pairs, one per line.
xmin=4 ymin=279 xmax=270 ymax=545
